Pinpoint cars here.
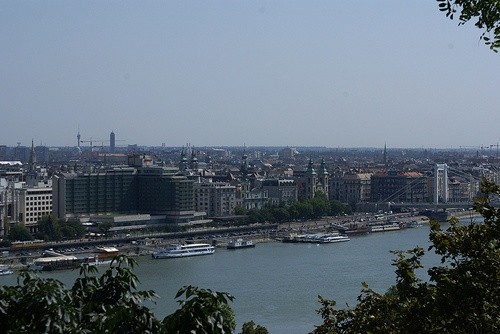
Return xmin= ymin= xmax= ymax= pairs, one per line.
xmin=53 ymin=246 xmax=92 ymax=254
xmin=186 ymin=231 xmax=255 ymax=241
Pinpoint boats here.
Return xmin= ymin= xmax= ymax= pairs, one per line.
xmin=226 ymin=238 xmax=256 ymax=248
xmin=370 ymin=223 xmax=401 ymax=232
xmin=0 ymin=266 xmax=13 ymax=275
xmin=276 ymin=231 xmax=351 ymax=243
xmin=33 ymin=248 xmax=79 ymax=269
xmin=152 ymin=244 xmax=215 ymax=258
